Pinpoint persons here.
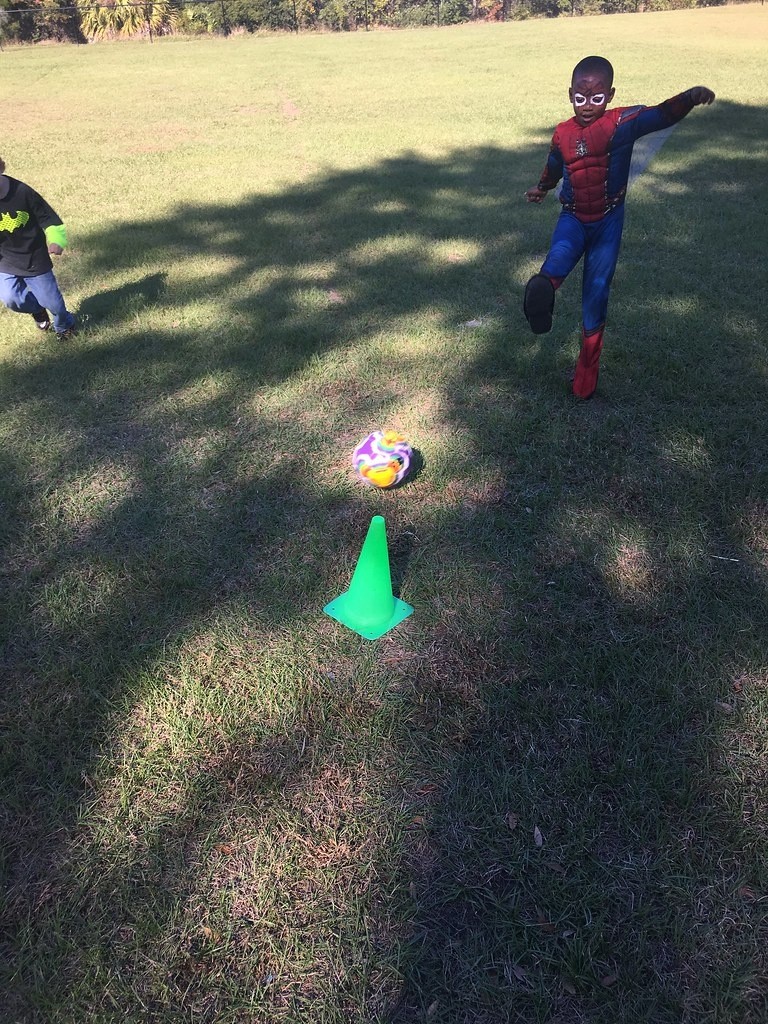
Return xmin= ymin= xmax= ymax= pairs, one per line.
xmin=523 ymin=56 xmax=715 ymax=401
xmin=0 ymin=157 xmax=76 ymax=343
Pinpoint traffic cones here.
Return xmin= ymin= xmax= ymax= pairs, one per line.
xmin=322 ymin=515 xmax=417 ymax=643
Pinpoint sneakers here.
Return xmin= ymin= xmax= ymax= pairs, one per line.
xmin=56 ymin=324 xmax=75 ymax=343
xmin=32 ymin=308 xmax=50 ymax=330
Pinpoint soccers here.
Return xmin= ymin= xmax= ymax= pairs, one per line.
xmin=351 ymin=430 xmax=414 ymax=490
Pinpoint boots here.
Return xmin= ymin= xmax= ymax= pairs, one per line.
xmin=524 ymin=271 xmax=565 ymax=334
xmin=573 ymin=323 xmax=605 ymax=399
